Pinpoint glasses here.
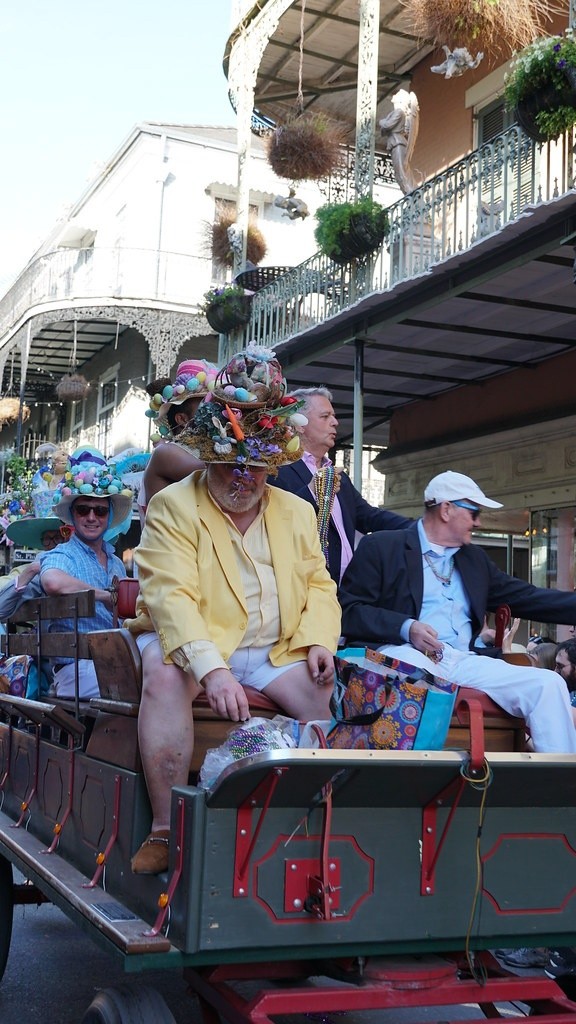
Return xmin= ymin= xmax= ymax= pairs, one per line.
xmin=452 ymin=501 xmax=481 ymax=520
xmin=529 ymin=637 xmax=544 ymax=643
xmin=40 ymin=535 xmax=64 ymax=547
xmin=73 ymin=505 xmax=109 ymax=517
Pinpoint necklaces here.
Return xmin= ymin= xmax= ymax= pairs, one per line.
xmin=314 ymin=467 xmax=337 ymax=568
xmin=424 ymin=555 xmax=455 ymax=583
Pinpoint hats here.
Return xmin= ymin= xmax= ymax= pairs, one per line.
xmin=182 ymin=358 xmax=304 ymax=466
xmin=52 ymin=460 xmax=134 ymax=529
xmin=424 ymin=470 xmax=504 ymax=509
xmin=6 ymin=462 xmax=65 ymax=550
xmin=146 ymin=360 xmax=221 ymax=447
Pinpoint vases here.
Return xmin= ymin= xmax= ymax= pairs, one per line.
xmin=56 ymin=382 xmax=85 ymax=401
xmin=329 ymin=212 xmax=384 ymax=265
xmin=206 ymin=295 xmax=251 ymax=333
xmin=514 ymin=65 xmax=576 ymax=142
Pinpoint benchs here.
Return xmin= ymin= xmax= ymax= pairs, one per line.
xmin=0 ymin=590 xmax=98 ymax=717
xmin=85 ymin=574 xmax=539 ymax=775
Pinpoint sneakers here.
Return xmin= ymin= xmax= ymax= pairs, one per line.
xmin=503 ymin=947 xmax=549 ymax=967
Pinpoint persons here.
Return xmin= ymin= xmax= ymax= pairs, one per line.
xmin=266 ymin=387 xmax=420 ymax=590
xmin=122 ymin=457 xmax=342 ymax=875
xmin=338 ymin=470 xmax=576 ymax=755
xmin=0 ymin=495 xmax=138 ymax=702
xmin=139 ymin=396 xmax=210 ymax=541
xmin=474 ymin=610 xmax=576 ymax=725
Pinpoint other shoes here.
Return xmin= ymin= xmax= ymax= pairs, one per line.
xmin=131 ymin=829 xmax=172 ymax=874
xmin=550 ymin=958 xmax=565 ymax=966
xmin=544 ymin=966 xmax=574 ymax=979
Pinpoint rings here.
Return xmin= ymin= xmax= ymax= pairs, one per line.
xmin=425 ymin=649 xmax=429 ymax=656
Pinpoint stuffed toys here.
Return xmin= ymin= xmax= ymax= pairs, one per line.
xmin=45 ymin=452 xmax=69 ymax=489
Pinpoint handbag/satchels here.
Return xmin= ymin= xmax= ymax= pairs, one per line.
xmin=116 ymin=577 xmax=140 ymax=619
xmin=326 ymin=648 xmax=460 ymax=751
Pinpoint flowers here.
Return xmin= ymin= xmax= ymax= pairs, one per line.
xmin=193 ymin=278 xmax=246 ymax=317
xmin=495 ymin=28 xmax=576 ymax=142
xmin=65 ymin=463 xmax=123 ymax=494
xmin=313 ymin=191 xmax=390 ymax=256
xmin=229 ymin=396 xmax=308 ymax=502
